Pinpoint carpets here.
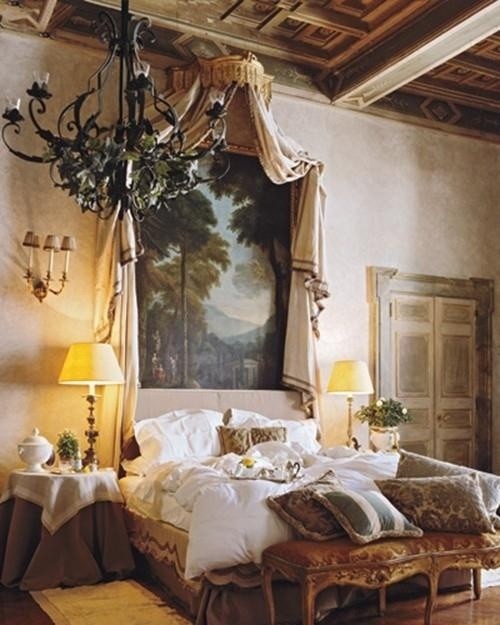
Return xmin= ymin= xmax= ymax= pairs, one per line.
xmin=27 ymin=579 xmax=195 ymax=625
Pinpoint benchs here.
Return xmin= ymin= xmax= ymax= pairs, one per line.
xmin=263 ymin=525 xmax=499 ymax=623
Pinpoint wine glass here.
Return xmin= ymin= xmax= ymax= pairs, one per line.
xmin=17 ymin=428 xmax=52 ymax=475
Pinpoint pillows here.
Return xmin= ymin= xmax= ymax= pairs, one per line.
xmin=396 ymin=449 xmax=500 ymax=515
xmin=311 ymin=486 xmax=423 ymax=543
xmin=133 ymin=405 xmax=322 ymax=461
xmin=373 ymin=472 xmax=496 ymax=534
xmin=267 ymin=471 xmax=352 ymax=541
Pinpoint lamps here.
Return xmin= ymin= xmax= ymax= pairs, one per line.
xmin=22 ymin=228 xmax=74 ymax=300
xmin=0 ymin=2 xmax=229 ymax=211
xmin=55 ymin=339 xmax=124 ymax=468
xmin=326 ymin=357 xmax=376 ymax=451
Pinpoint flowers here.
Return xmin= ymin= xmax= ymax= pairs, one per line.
xmin=353 ymin=398 xmax=415 ymax=426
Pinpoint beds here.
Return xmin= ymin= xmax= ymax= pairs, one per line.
xmin=114 ymin=420 xmax=500 ymax=621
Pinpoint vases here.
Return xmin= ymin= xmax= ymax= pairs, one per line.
xmin=370 ymin=425 xmax=400 ymax=452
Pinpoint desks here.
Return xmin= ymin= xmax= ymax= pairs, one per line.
xmin=8 ymin=468 xmax=132 ymax=590
